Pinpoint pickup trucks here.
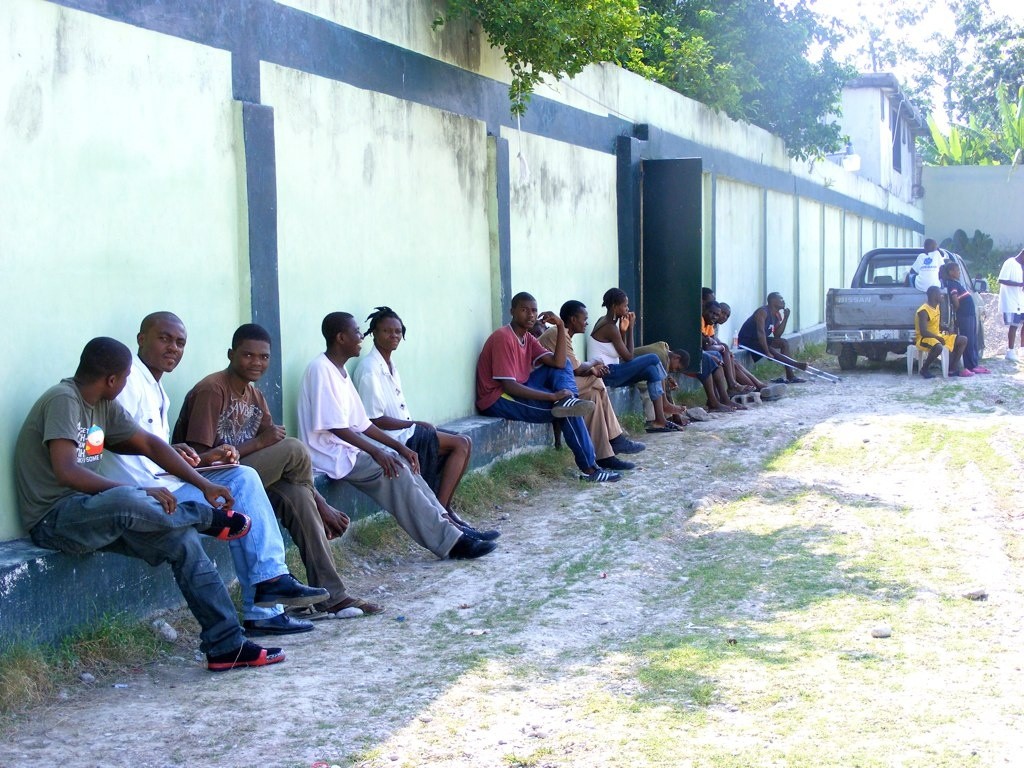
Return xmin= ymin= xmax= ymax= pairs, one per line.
xmin=825 ymin=247 xmax=989 ymax=371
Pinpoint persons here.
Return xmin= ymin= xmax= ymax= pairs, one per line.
xmin=476 ymin=292 xmax=622 ymax=483
xmin=906 ymin=239 xmax=1024 ymax=378
xmin=300 ymin=313 xmax=499 ymax=559
xmin=17 ymin=337 xmax=286 ymax=671
xmin=353 ymin=308 xmax=474 ymax=531
xmin=533 ymin=287 xmax=810 ymax=471
xmin=115 ymin=312 xmax=332 ymax=636
xmin=170 ymin=323 xmax=388 ymax=616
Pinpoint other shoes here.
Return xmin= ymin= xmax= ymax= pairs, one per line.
xmin=1005 ymin=349 xmax=1020 ymax=362
xmin=1019 ymin=347 xmax=1024 ymax=357
xmin=959 ymin=368 xmax=974 ymax=377
xmin=760 ymin=383 xmax=787 ymax=398
xmin=791 ymin=376 xmax=808 ymax=383
xmin=449 ymin=511 xmax=476 ymax=531
xmin=448 ymin=536 xmax=498 ymax=558
xmin=919 ymin=369 xmax=937 ymax=378
xmin=461 ymin=525 xmax=500 ymax=540
xmin=972 ymin=367 xmax=991 ymax=373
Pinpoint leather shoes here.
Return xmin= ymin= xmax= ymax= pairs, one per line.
xmin=242 ymin=614 xmax=316 ymax=636
xmin=254 ymin=573 xmax=330 ymax=607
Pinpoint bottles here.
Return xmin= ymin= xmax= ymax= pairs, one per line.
xmin=732 ymin=329 xmax=739 ymax=349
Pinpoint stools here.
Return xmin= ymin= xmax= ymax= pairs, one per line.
xmin=907 ymin=345 xmax=949 ymax=379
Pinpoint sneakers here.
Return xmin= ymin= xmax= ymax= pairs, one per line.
xmin=207 ymin=638 xmax=285 ymax=669
xmin=596 ymin=454 xmax=635 ymax=470
xmin=611 ymin=435 xmax=646 ymax=455
xmin=204 ymin=508 xmax=252 ymax=540
xmin=550 ymin=395 xmax=595 ymax=418
xmin=580 ymin=469 xmax=622 ymax=482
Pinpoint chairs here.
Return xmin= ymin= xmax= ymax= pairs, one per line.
xmin=873 ymin=276 xmax=893 ymax=284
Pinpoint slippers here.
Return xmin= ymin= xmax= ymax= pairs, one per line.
xmin=660 ymin=422 xmax=684 ymax=431
xmin=645 ymin=427 xmax=667 ymax=433
xmin=334 ymin=597 xmax=387 ymax=615
xmin=739 ymin=385 xmax=756 ymax=394
